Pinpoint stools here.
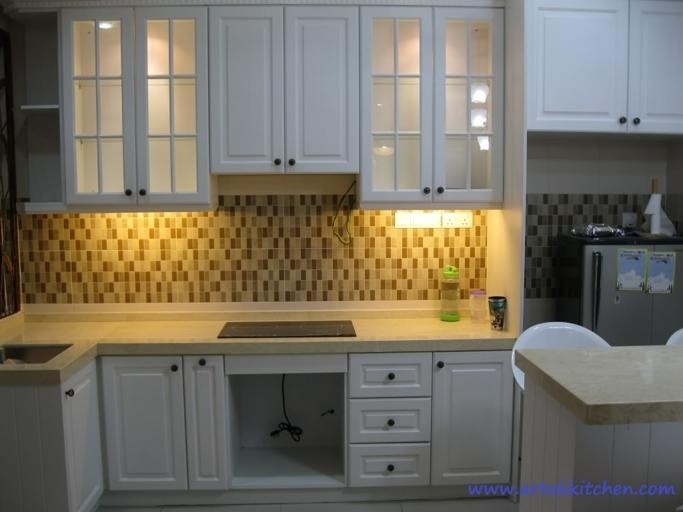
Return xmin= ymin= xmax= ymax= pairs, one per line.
xmin=509 ymin=319 xmax=611 ymax=392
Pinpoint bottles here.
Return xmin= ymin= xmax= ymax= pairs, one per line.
xmin=468 ymin=289 xmax=486 ymax=321
xmin=439 ymin=265 xmax=461 ymax=322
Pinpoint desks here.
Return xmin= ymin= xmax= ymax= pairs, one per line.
xmin=509 ymin=343 xmax=683 ymax=512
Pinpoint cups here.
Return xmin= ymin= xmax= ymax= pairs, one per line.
xmin=487 ymin=296 xmax=506 ymax=331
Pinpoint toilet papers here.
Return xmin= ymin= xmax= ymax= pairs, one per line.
xmin=644 ymin=193 xmax=676 ymax=237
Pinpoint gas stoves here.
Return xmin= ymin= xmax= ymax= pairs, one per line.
xmin=215 ymin=318 xmax=358 ymax=340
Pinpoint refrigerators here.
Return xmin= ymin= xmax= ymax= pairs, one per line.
xmin=558 ymin=230 xmax=683 ymax=346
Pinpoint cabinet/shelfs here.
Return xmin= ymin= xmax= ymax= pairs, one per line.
xmin=349 ymin=348 xmax=430 ymax=490
xmin=57 ymin=3 xmax=220 ymax=213
xmin=493 ymin=0 xmax=683 ymax=136
xmin=432 ymin=350 xmax=516 ymax=487
xmin=181 ymin=354 xmax=243 ymax=489
xmin=206 ymin=2 xmax=360 ymax=183
xmin=355 ymin=1 xmax=503 ymax=210
xmin=97 ymin=356 xmax=189 ymax=492
xmin=2 ymin=360 xmax=107 ymax=512
xmin=10 ymin=6 xmax=64 ymax=214
